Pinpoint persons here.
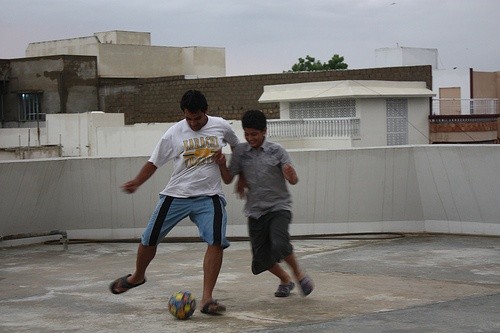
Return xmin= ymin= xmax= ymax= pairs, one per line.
xmin=211 ymin=110 xmax=317 ymax=298
xmin=109 ymin=89 xmax=248 ymax=317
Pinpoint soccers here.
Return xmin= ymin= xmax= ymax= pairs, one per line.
xmin=167 ymin=291 xmax=197 ymax=321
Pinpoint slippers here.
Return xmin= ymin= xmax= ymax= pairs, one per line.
xmin=200 ymin=300 xmax=226 ymax=315
xmin=110 ymin=274 xmax=145 ymax=294
xmin=299 ymin=276 xmax=314 ymax=295
xmin=275 ymin=282 xmax=294 ymax=297
